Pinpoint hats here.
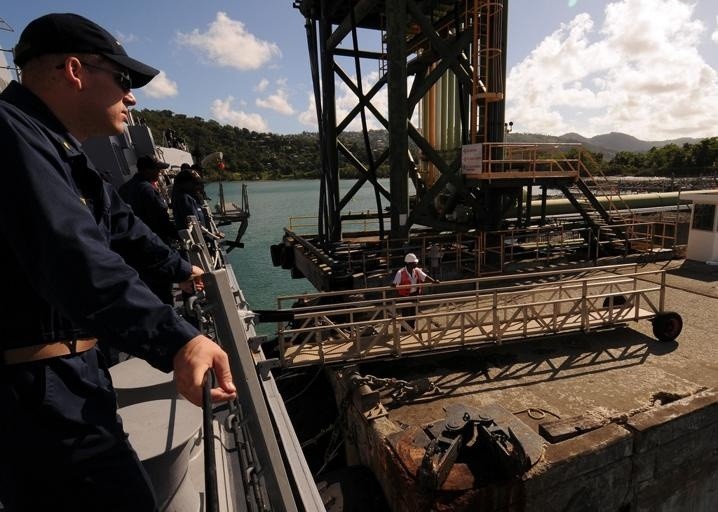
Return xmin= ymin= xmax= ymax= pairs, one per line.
xmin=137 ymin=153 xmax=169 ymax=172
xmin=14 ymin=13 xmax=160 ymax=90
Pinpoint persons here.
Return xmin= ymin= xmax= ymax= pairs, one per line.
xmin=391 ymin=253 xmax=441 ymax=333
xmin=0 ymin=10 xmax=241 ymax=510
xmin=113 ymin=126 xmax=213 ymax=310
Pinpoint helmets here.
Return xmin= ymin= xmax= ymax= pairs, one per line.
xmin=404 ymin=253 xmax=419 ymax=263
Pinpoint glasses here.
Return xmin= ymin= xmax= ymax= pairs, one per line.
xmin=55 ymin=58 xmax=132 ymax=92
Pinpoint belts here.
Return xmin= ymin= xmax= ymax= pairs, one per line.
xmin=5 ymin=338 xmax=97 ymax=365
